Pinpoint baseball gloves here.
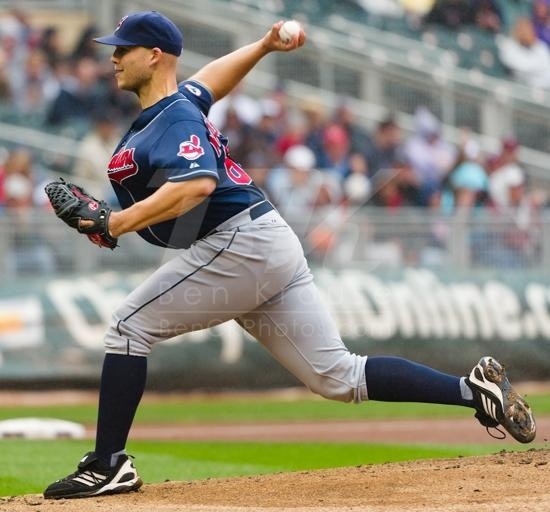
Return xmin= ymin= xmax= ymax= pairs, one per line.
xmin=44 ymin=177 xmax=120 ymax=251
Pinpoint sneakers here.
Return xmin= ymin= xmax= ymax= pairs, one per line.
xmin=464 ymin=353 xmax=536 ymax=446
xmin=43 ymin=448 xmax=142 ymax=498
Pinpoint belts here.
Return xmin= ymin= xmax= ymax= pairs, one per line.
xmin=199 ymin=201 xmax=276 ymax=239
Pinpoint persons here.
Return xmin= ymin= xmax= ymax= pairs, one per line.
xmin=41 ymin=8 xmax=539 ymax=501
xmin=0 ymin=0 xmax=550 ymax=270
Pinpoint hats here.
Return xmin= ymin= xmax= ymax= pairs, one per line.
xmin=90 ymin=10 xmax=182 ymax=57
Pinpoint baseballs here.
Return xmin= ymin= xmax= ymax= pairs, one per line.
xmin=279 ymin=19 xmax=301 ymax=44
